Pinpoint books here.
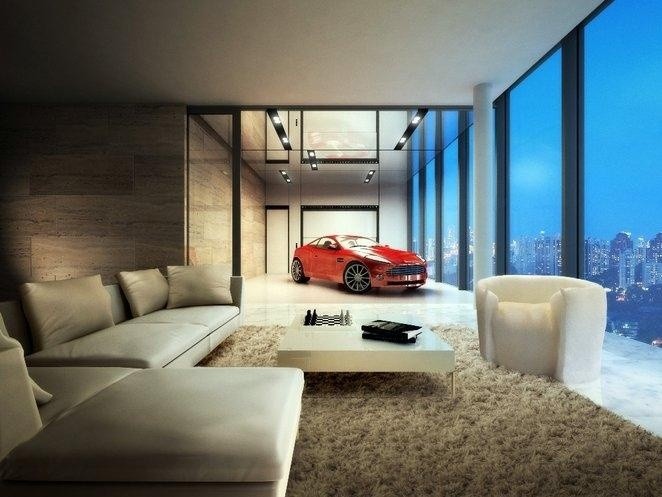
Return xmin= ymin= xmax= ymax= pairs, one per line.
xmin=362 ymin=320 xmax=423 ymax=343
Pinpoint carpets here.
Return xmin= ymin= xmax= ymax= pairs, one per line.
xmin=195 ymin=326 xmax=662 ymax=497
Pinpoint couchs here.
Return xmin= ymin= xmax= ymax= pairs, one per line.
xmin=476 ymin=275 xmax=607 ymax=384
xmin=168 ymin=266 xmax=232 ymax=308
xmin=0 ymin=347 xmax=305 ymax=497
xmin=0 ymin=277 xmax=246 ymax=369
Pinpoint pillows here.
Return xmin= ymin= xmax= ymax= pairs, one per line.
xmin=20 ymin=274 xmax=114 ymax=351
xmin=117 ymin=269 xmax=169 ymax=317
xmin=0 ymin=315 xmax=53 ymax=404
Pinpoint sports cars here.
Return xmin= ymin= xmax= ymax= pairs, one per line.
xmin=291 ymin=234 xmax=428 ymax=295
